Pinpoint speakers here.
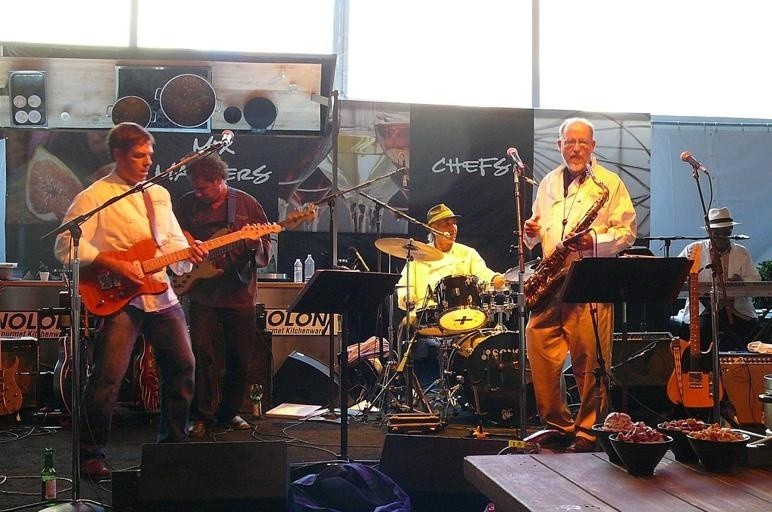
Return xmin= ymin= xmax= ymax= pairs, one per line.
xmin=134 ymin=438 xmax=292 ymax=508
xmin=376 ymin=433 xmax=519 ymax=498
xmin=274 ymin=350 xmax=340 ymax=409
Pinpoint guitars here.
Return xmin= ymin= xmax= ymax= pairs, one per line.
xmin=53 ymin=272 xmax=161 ymax=425
xmin=79 ymin=222 xmax=282 ymax=314
xmin=0 ymin=281 xmax=23 ymax=416
xmin=666 ymin=244 xmax=724 ymax=408
xmin=166 ymin=202 xmax=317 ymax=296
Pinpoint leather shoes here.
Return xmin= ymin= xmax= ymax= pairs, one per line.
xmin=78 ymin=458 xmax=112 ymax=482
xmin=565 ymin=433 xmax=597 ymax=454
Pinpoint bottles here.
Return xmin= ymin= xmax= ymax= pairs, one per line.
xmin=40 ymin=447 xmax=58 ymax=507
xmin=293 ymin=258 xmax=303 ymax=283
xmin=251 ymin=383 xmax=263 ymax=420
xmin=305 ymin=254 xmax=315 ymax=282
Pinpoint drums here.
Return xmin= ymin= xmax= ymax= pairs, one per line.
xmin=480 ymin=290 xmax=521 ymax=314
xmin=415 ymin=307 xmax=442 ymax=329
xmin=443 ymin=328 xmax=542 ymax=429
xmin=433 ymin=274 xmax=489 ymax=333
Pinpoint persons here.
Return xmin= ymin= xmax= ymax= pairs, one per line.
xmin=54 ymin=122 xmax=196 ymax=481
xmin=677 ymin=207 xmax=766 ymax=352
xmin=173 ymin=152 xmax=274 ymax=437
xmin=523 ymin=118 xmax=637 ymax=453
xmin=396 ymin=203 xmax=506 ymax=399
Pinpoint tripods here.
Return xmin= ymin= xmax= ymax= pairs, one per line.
xmin=354 ymin=315 xmax=451 ymax=423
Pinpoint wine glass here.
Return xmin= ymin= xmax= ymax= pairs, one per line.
xmin=280 ymin=101 xmax=413 ymax=233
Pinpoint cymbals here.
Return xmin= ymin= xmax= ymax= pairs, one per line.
xmin=503 ymin=258 xmax=543 ymax=284
xmin=375 ymin=238 xmax=444 ymax=262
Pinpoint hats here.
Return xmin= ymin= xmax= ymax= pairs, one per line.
xmin=425 ymin=203 xmax=460 ymax=230
xmin=700 ymin=206 xmax=741 ymax=230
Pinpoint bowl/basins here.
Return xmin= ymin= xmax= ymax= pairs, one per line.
xmin=592 ymin=420 xmax=750 ymax=477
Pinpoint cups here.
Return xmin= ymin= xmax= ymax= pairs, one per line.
xmin=40 ymin=270 xmax=49 ymax=281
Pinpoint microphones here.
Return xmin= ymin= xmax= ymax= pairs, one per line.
xmin=676 ymin=150 xmax=710 ymax=179
xmin=220 ymin=126 xmax=235 ymax=150
xmin=506 ymin=145 xmax=529 ymax=173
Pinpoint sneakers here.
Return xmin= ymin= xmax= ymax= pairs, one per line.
xmin=220 ymin=409 xmax=251 ymax=432
xmin=182 ymin=415 xmax=206 ymax=438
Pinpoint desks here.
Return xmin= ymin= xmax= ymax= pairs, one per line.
xmin=462 ymin=442 xmax=772 ymax=511
xmin=256 ymin=282 xmax=342 ymax=382
xmin=0 ymin=279 xmax=72 ymax=312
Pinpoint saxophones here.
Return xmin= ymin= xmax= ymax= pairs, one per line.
xmin=524 ymin=161 xmax=609 ymax=313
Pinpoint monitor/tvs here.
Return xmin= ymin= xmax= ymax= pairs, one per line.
xmin=112 ymin=64 xmax=213 ymax=134
